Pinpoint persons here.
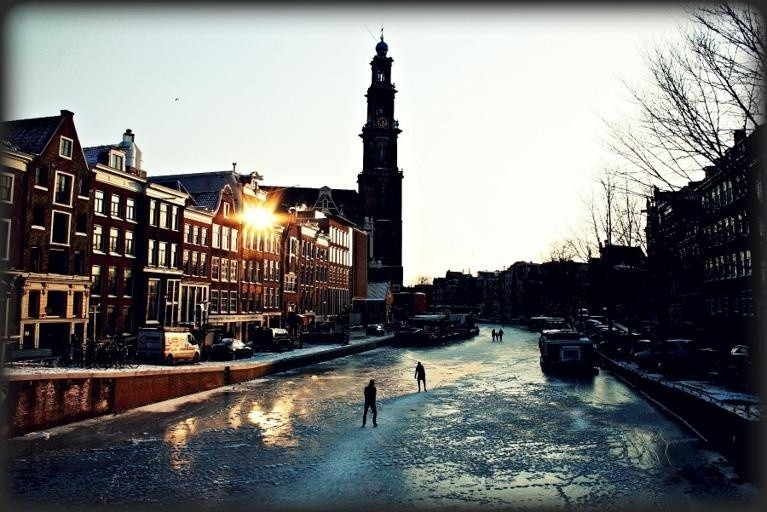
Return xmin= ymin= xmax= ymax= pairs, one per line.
xmin=498 ymin=328 xmax=504 ymax=342
xmin=414 ymin=362 xmax=427 ymax=393
xmin=360 ymin=379 xmax=378 ymax=427
xmin=69 ymin=334 xmax=84 ymax=369
xmin=491 ymin=328 xmax=496 ymax=342
xmin=495 ymin=332 xmax=499 ymax=342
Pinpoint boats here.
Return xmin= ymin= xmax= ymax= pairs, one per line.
xmin=528 ymin=316 xmax=567 ymax=332
xmin=538 ymin=328 xmax=595 ymax=379
xmin=405 ymin=310 xmax=479 ymax=346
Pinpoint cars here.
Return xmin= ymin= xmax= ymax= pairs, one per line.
xmin=577 ymin=308 xmax=695 ymax=366
xmin=207 ymin=337 xmax=253 ymax=360
xmin=366 ymin=323 xmax=385 ymax=336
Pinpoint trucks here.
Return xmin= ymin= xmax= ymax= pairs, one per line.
xmin=253 ymin=326 xmax=294 ymax=352
xmin=137 ymin=326 xmax=201 ymax=366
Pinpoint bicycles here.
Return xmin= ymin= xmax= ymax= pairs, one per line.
xmin=65 ymin=335 xmax=141 ymax=369
